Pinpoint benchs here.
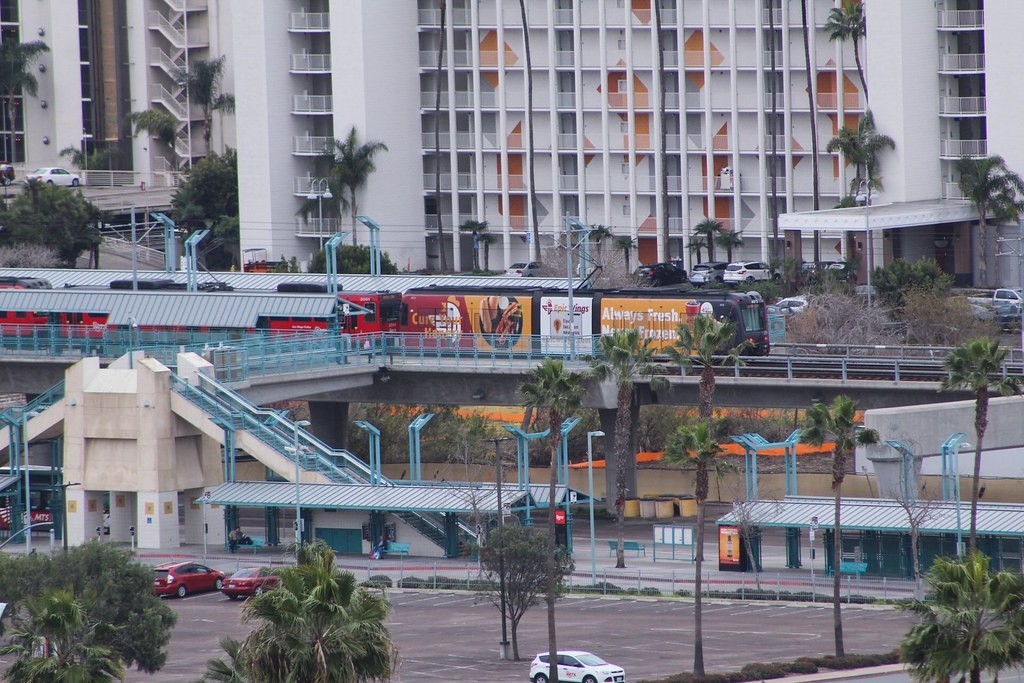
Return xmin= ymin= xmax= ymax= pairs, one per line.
xmin=608 ymin=540 xmax=646 ymax=559
xmin=373 ymin=541 xmax=410 ymax=561
xmin=226 ymin=534 xmax=264 ymax=555
xmin=830 ymin=561 xmax=868 ymax=579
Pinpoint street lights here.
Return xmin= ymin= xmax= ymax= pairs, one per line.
xmin=308 ymin=178 xmax=333 ymax=253
xmin=128 ymin=312 xmax=137 ymax=368
xmin=294 ymin=420 xmax=311 ymax=567
xmin=23 ymin=411 xmax=39 ymax=556
xmin=855 ymin=179 xmax=879 ymax=309
xmin=587 ymin=430 xmax=606 ymax=587
xmin=955 ymin=441 xmax=972 ymax=562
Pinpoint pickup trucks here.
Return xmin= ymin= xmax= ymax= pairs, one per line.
xmin=967 ymin=288 xmax=1024 ymax=316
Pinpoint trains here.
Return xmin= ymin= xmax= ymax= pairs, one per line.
xmin=0 ymin=276 xmax=769 ymax=362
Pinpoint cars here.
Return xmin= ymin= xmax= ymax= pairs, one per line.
xmin=503 ymin=262 xmax=550 ymax=278
xmin=222 ymin=566 xmax=281 ymax=599
xmin=774 ymin=262 xmax=850 ymax=284
xmin=0 ymin=161 xmax=80 ymax=187
xmin=529 ymin=651 xmax=625 ymax=683
xmin=775 ymin=297 xmax=810 ymax=315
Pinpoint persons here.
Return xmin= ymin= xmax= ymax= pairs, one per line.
xmin=229 ymin=526 xmax=246 ymax=554
xmin=373 ymin=534 xmax=389 ymax=560
xmin=363 ymin=336 xmax=373 ymax=364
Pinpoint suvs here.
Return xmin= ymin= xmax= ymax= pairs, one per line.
xmin=723 ymin=261 xmax=778 ymax=287
xmin=633 ymin=263 xmax=687 ymax=287
xmin=150 ymin=561 xmax=225 ymax=598
xmin=688 ymin=262 xmax=728 ymax=286
